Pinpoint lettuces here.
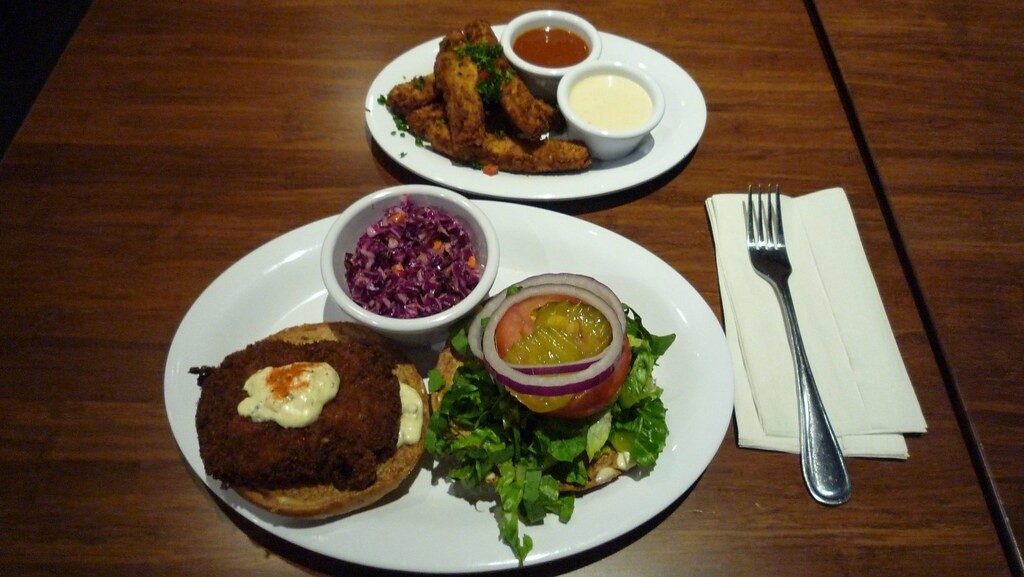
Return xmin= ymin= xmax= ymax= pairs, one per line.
xmin=423 ymin=288 xmax=676 ymax=573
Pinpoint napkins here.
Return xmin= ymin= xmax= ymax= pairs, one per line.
xmin=701 ymin=179 xmax=933 ymax=463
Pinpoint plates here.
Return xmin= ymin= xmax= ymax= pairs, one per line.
xmin=363 ymin=30 xmax=709 ymax=202
xmin=162 ymin=200 xmax=738 ymax=575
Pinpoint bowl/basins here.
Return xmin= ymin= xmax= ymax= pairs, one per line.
xmin=318 ymin=183 xmax=499 ymax=343
xmin=556 ymin=63 xmax=665 ymax=160
xmin=501 ymin=10 xmax=601 ymax=100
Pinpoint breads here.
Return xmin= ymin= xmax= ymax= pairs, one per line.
xmin=226 ymin=322 xmax=427 ymax=518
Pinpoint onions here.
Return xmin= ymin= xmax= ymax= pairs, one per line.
xmin=344 ymin=195 xmax=476 ymax=320
xmin=465 ymin=273 xmax=626 ymax=396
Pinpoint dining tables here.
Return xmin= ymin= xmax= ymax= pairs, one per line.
xmin=1 ymin=0 xmax=1022 ymax=573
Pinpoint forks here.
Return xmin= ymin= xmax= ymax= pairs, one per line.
xmin=746 ymin=180 xmax=854 ymax=508
